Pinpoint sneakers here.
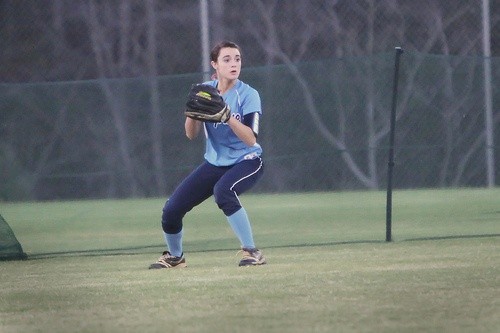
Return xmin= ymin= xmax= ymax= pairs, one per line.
xmin=238 ymin=248 xmax=266 ymax=266
xmin=147 ymin=253 xmax=186 ymax=270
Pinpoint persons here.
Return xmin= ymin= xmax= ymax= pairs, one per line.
xmin=148 ymin=42 xmax=268 ymax=270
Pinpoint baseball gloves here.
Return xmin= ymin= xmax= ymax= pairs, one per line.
xmin=185 ymin=84 xmax=232 ymax=124
